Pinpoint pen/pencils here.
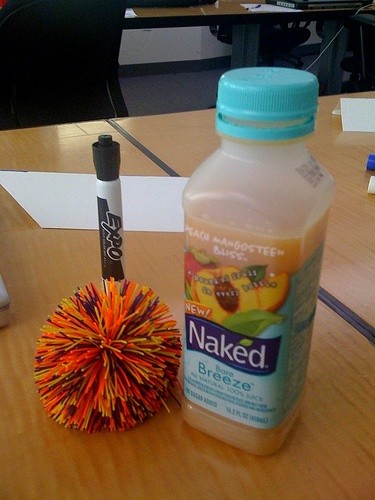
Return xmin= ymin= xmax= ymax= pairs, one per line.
xmin=245 ymin=4 xmax=261 ymax=10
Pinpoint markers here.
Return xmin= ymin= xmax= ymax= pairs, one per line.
xmin=91 ymin=133 xmax=127 ymax=294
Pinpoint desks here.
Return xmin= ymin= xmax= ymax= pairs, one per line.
xmin=0 ymin=89 xmax=375 ymax=497
xmin=123 ymin=0 xmax=375 ymax=69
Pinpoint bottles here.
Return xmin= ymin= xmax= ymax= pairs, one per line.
xmin=179 ymin=66 xmax=333 ymax=461
